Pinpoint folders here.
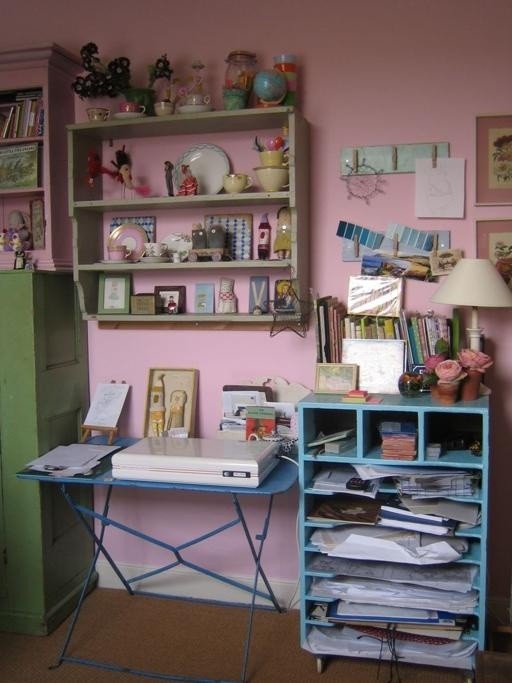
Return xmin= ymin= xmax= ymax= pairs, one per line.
xmin=376 ymin=510 xmax=458 ymax=537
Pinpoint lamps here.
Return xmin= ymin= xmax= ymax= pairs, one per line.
xmin=431 ymin=258 xmax=511 ymax=394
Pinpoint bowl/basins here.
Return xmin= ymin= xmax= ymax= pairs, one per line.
xmin=252 ymin=167 xmax=290 ymax=190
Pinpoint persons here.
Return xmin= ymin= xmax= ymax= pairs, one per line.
xmin=165 ymin=160 xmax=198 ymax=197
xmin=2 ymin=225 xmax=35 ymax=271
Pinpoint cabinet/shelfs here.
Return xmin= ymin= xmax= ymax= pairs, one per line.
xmin=0 ymin=42 xmax=93 ymax=268
xmin=2 ymin=269 xmax=97 ymax=639
xmin=297 ymin=393 xmax=489 ymax=679
xmin=67 ymin=111 xmax=313 ymax=324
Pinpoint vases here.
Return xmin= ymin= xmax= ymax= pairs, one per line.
xmin=462 ymin=369 xmax=481 ymax=399
xmin=433 ymin=382 xmax=461 ymax=404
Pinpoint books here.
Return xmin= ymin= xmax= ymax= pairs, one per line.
xmin=219 ymin=385 xmax=298 ymax=441
xmin=314 ymin=275 xmax=479 ymax=395
xmin=304 ymin=420 xmax=479 ymax=640
xmin=2 ymin=88 xmax=45 ymax=189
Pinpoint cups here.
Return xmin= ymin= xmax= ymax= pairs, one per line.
xmin=221 ymin=171 xmax=254 ymax=193
xmin=118 ymin=102 xmax=146 ymax=113
xmin=140 ymin=240 xmax=169 ymax=255
xmin=105 ymin=243 xmax=132 ymax=260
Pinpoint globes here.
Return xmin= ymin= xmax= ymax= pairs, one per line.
xmin=252 ymin=67 xmax=287 ymax=108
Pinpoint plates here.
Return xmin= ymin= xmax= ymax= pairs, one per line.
xmin=175 ymin=104 xmax=217 ymax=114
xmin=97 ymin=259 xmax=135 ymax=263
xmin=171 ymin=143 xmax=232 ymax=194
xmin=106 ymin=221 xmax=150 ymax=261
xmin=112 ymin=110 xmax=147 ymax=119
xmin=137 ymin=256 xmax=170 ymax=262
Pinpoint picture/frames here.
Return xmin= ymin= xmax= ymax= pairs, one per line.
xmin=475 ymin=217 xmax=512 ymax=290
xmin=314 ymin=362 xmax=359 ymax=395
xmin=473 ymin=114 xmax=512 ymax=206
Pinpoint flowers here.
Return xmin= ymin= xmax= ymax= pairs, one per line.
xmin=456 ymin=348 xmax=496 ymax=373
xmin=435 ymin=359 xmax=466 ymax=383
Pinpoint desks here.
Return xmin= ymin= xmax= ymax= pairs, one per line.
xmin=19 ymin=434 xmax=299 ymax=682
